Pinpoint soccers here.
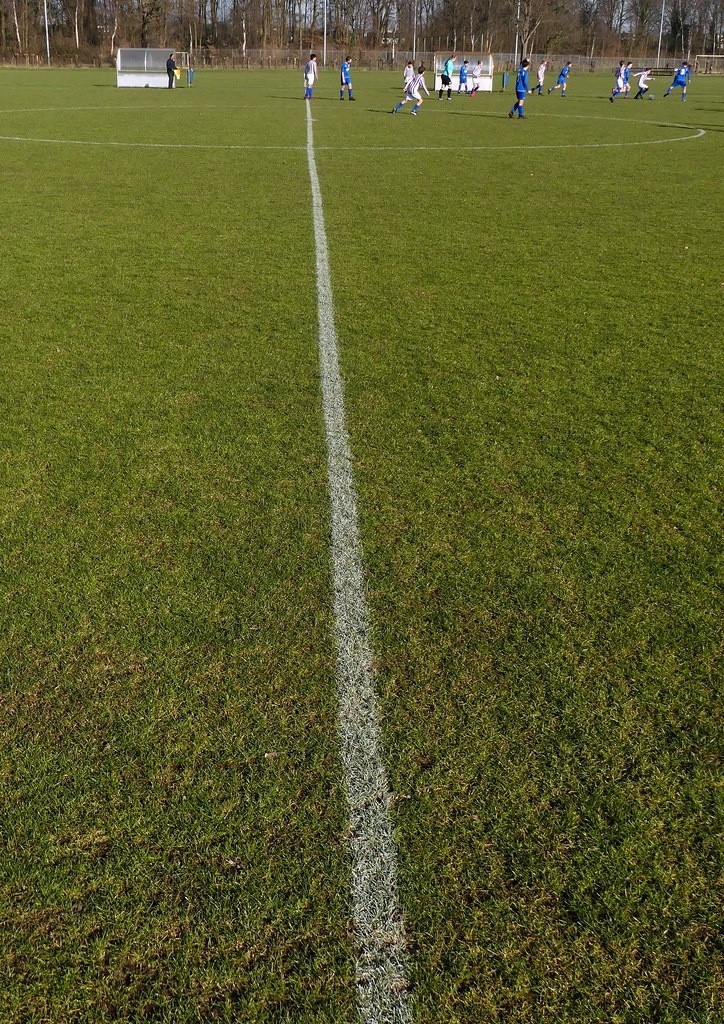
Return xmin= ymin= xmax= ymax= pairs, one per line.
xmin=649 ymin=94 xmax=654 ymax=100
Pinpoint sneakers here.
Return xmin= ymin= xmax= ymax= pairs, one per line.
xmin=447 ymin=97 xmax=453 ymax=100
xmin=469 ymin=93 xmax=476 ymax=98
xmin=561 ymin=93 xmax=567 ymax=98
xmin=350 ymin=97 xmax=356 ymax=100
xmin=438 ymin=98 xmax=444 ymax=101
xmin=392 ymin=108 xmax=397 ymax=115
xmin=538 ymin=94 xmax=544 ymax=97
xmin=412 ymin=110 xmax=418 ymax=116
xmin=548 ymin=88 xmax=551 ymax=95
xmin=640 ymin=93 xmax=644 ymax=98
xmin=634 ymin=95 xmax=639 ymax=100
xmin=608 ymin=97 xmax=615 ymax=104
xmin=532 ymin=88 xmax=534 ymax=95
xmin=664 ymin=93 xmax=669 ymax=97
xmin=518 ymin=115 xmax=528 ymax=120
xmin=509 ymin=112 xmax=514 ymax=118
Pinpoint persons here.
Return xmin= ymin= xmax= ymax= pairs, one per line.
xmin=167 ymin=54 xmax=179 ymax=88
xmin=609 ymin=61 xmax=692 ymax=104
xmin=304 ymin=54 xmax=319 ymax=99
xmin=392 ymin=54 xmax=482 ymax=116
xmin=340 ymin=56 xmax=356 ymax=101
xmin=506 ymin=57 xmax=572 ymax=120
